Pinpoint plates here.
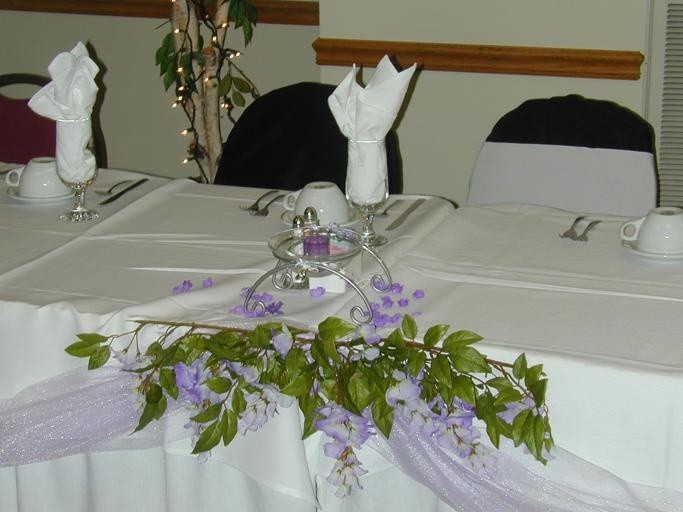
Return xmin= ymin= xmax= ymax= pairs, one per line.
xmin=5 ymin=187 xmax=77 ymax=206
xmin=618 ymin=241 xmax=683 ymax=263
xmin=279 ymin=210 xmax=362 ymax=230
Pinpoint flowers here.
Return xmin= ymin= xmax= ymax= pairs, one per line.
xmin=65 ymin=310 xmax=553 ymax=497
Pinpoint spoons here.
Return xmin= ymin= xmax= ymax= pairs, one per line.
xmin=560 ymin=214 xmax=603 ymax=243
xmin=236 ymin=189 xmax=288 ymax=219
xmin=93 ymin=180 xmax=141 ymax=195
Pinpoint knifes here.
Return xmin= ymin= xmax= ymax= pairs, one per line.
xmin=98 ymin=178 xmax=149 ymax=206
xmin=385 ymin=197 xmax=427 ymax=231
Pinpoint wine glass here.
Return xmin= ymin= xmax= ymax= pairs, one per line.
xmin=54 ymin=115 xmax=102 ymax=225
xmin=343 ymin=142 xmax=389 ymax=252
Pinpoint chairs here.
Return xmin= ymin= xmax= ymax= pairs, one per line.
xmin=1 ymin=72 xmax=111 ymax=171
xmin=466 ymin=91 xmax=659 ymax=213
xmin=211 ymin=79 xmax=406 ymax=196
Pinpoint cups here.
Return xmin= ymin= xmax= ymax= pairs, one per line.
xmin=621 ymin=205 xmax=682 ymax=256
xmin=284 ymin=182 xmax=353 ymax=229
xmin=6 ymin=156 xmax=74 ymax=199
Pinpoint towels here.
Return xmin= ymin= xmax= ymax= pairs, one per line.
xmin=326 ymin=50 xmax=420 ymax=204
xmin=26 ymin=39 xmax=99 ymax=182
